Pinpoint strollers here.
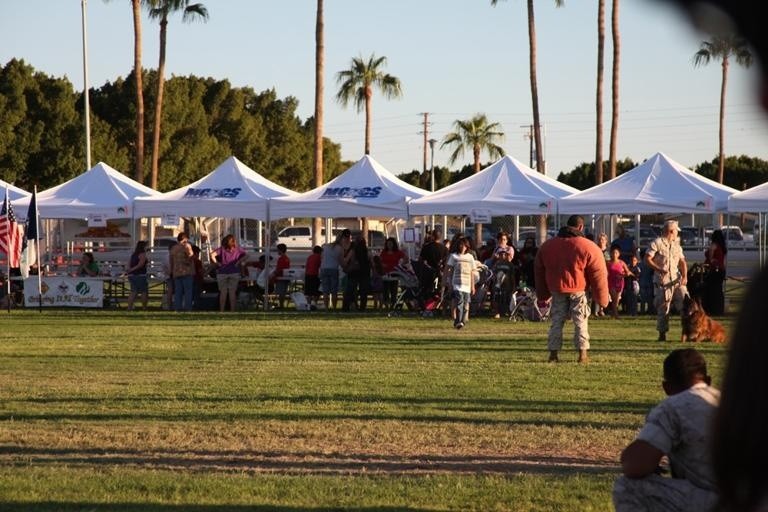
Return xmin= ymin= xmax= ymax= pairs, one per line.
xmin=380 ymin=257 xmax=439 ymax=320
xmin=508 ymin=286 xmax=552 ymax=323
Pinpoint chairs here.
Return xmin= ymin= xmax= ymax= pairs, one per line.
xmin=34 ymin=264 xmax=544 ymax=322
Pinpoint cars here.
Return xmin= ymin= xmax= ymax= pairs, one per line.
xmin=144 ymin=236 xmax=179 ymax=274
xmin=618 ymin=220 xmax=758 ymax=252
xmin=347 ymin=230 xmax=386 ymax=251
xmin=416 ymin=221 xmax=564 ymax=253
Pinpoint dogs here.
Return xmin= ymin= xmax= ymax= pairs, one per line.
xmin=680 ymin=293 xmax=725 ymax=344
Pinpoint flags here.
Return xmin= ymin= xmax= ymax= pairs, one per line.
xmin=20 ymin=188 xmax=45 ymax=280
xmin=0 ymin=194 xmax=22 ymax=269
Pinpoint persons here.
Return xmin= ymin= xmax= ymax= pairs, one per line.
xmin=122 ymin=213 xmax=732 ymax=364
xmin=77 ymin=253 xmax=98 ymax=276
xmin=705 ymin=229 xmax=727 ymax=316
xmin=242 ymin=242 xmax=291 ymax=308
xmin=584 ymin=226 xmax=654 ymax=318
xmin=477 ymin=232 xmax=539 ymax=319
xmin=418 ymin=228 xmax=480 ymax=329
xmin=166 ymin=231 xmax=204 ymax=313
xmin=645 ymin=220 xmax=690 ymax=341
xmin=303 ymin=229 xmax=405 ymax=313
xmin=210 ymin=234 xmax=248 ymax=312
xmin=126 ymin=241 xmax=148 ymax=310
xmin=534 ymin=215 xmax=609 ymax=362
xmin=613 ymin=348 xmax=721 ymax=512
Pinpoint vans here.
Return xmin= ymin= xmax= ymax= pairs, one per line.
xmin=273 ymin=225 xmax=353 ymax=251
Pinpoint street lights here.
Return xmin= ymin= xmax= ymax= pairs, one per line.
xmin=426 ymin=138 xmax=439 ymax=234
